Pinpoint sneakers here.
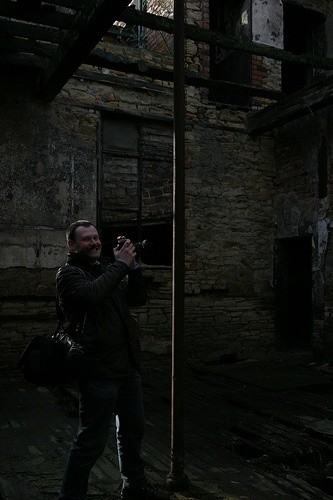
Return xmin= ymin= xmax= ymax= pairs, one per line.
xmin=121 ymin=479 xmax=170 ymax=500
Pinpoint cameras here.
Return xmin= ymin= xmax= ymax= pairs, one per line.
xmin=112 ymin=239 xmax=151 ymax=250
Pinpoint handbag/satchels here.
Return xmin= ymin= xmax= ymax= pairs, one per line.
xmin=17 ymin=335 xmax=83 ymax=388
xmin=47 ymin=328 xmax=86 ymax=370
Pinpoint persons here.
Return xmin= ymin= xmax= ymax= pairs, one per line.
xmin=55 ymin=219 xmax=170 ymax=500
